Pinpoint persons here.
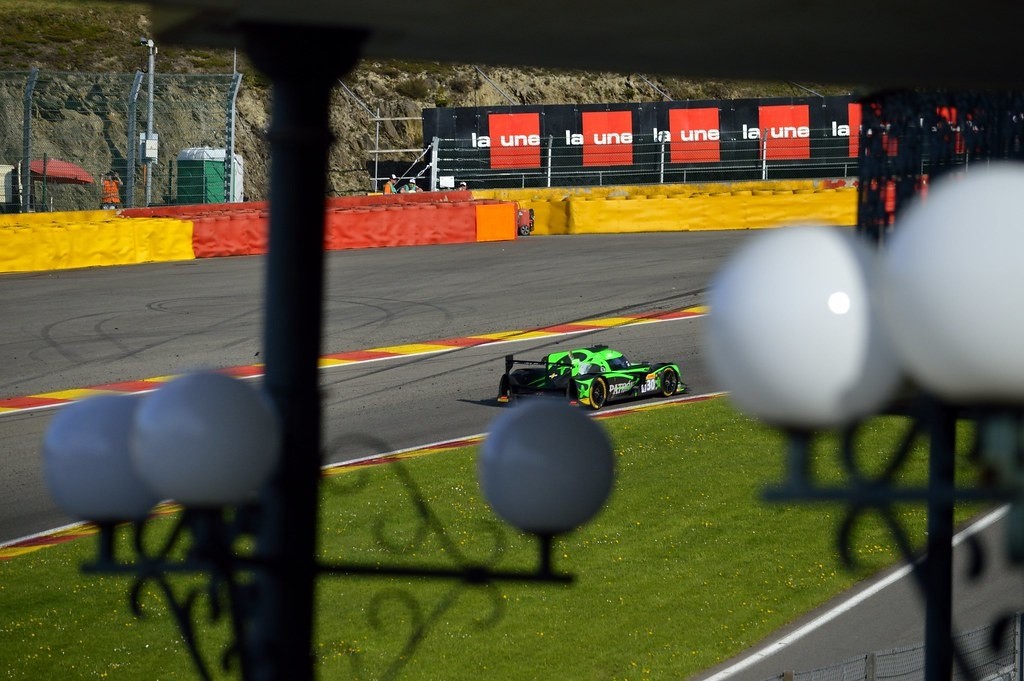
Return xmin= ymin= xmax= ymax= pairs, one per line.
xmin=383 ymin=174 xmax=399 ymax=195
xmin=99 ymin=171 xmax=123 ymax=209
xmin=400 ymin=179 xmax=423 ymax=193
xmin=458 ymin=182 xmax=466 ymax=191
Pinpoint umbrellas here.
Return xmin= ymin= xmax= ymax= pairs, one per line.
xmin=14 ymin=158 xmax=94 ymax=211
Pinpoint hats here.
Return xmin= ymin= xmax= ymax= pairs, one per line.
xmin=105 ymin=170 xmax=115 ymax=177
